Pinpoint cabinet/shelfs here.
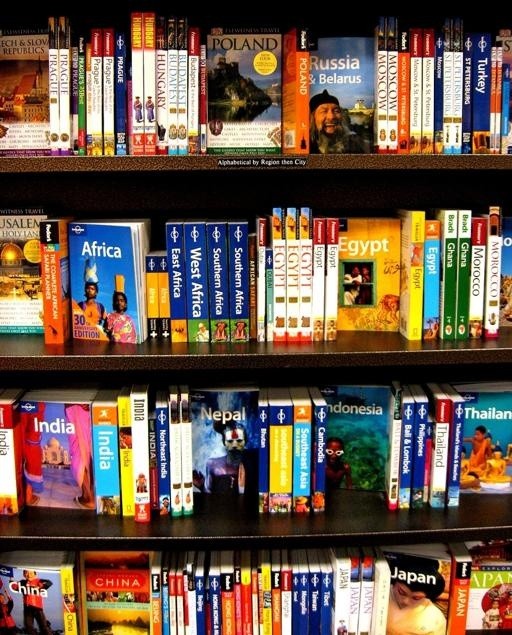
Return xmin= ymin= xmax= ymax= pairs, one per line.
xmin=0 ymin=154 xmax=512 ymax=552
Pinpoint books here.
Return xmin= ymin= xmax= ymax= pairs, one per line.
xmin=1 ymin=15 xmax=511 ymax=157
xmin=2 ymin=548 xmax=512 ymax=634
xmin=0 ymin=212 xmax=509 ymax=343
xmin=0 ymin=382 xmax=511 ymax=515
